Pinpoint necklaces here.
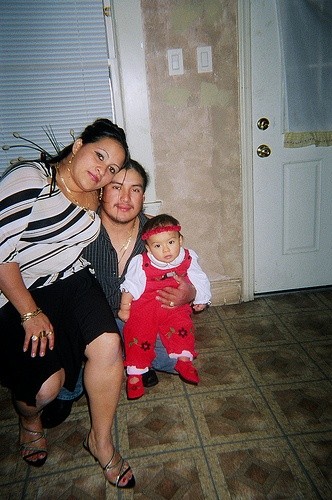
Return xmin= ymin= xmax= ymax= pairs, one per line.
xmin=56 ymin=162 xmax=96 ymax=222
xmin=117 ymin=217 xmax=136 ymax=264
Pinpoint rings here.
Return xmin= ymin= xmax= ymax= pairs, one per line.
xmin=31 ymin=335 xmax=39 ymax=341
xmin=46 ymin=331 xmax=54 ymax=336
xmin=39 ymin=331 xmax=47 ymax=337
xmin=170 ymin=302 xmax=175 ymax=307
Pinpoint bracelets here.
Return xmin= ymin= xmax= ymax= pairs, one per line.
xmin=21 ymin=308 xmax=42 ymax=323
xmin=121 ymin=302 xmax=132 ymax=307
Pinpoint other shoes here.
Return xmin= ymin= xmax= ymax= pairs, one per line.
xmin=40 ymin=390 xmax=86 ymax=429
xmin=141 ymin=369 xmax=158 ymax=387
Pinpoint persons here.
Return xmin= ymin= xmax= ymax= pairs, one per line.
xmin=119 ymin=213 xmax=212 ymax=399
xmin=0 ymin=118 xmax=165 ymax=489
xmin=40 ymin=159 xmax=179 ymax=429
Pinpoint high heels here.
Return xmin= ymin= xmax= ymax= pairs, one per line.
xmin=16 ymin=415 xmax=48 ymax=468
xmin=82 ymin=429 xmax=136 ymax=489
